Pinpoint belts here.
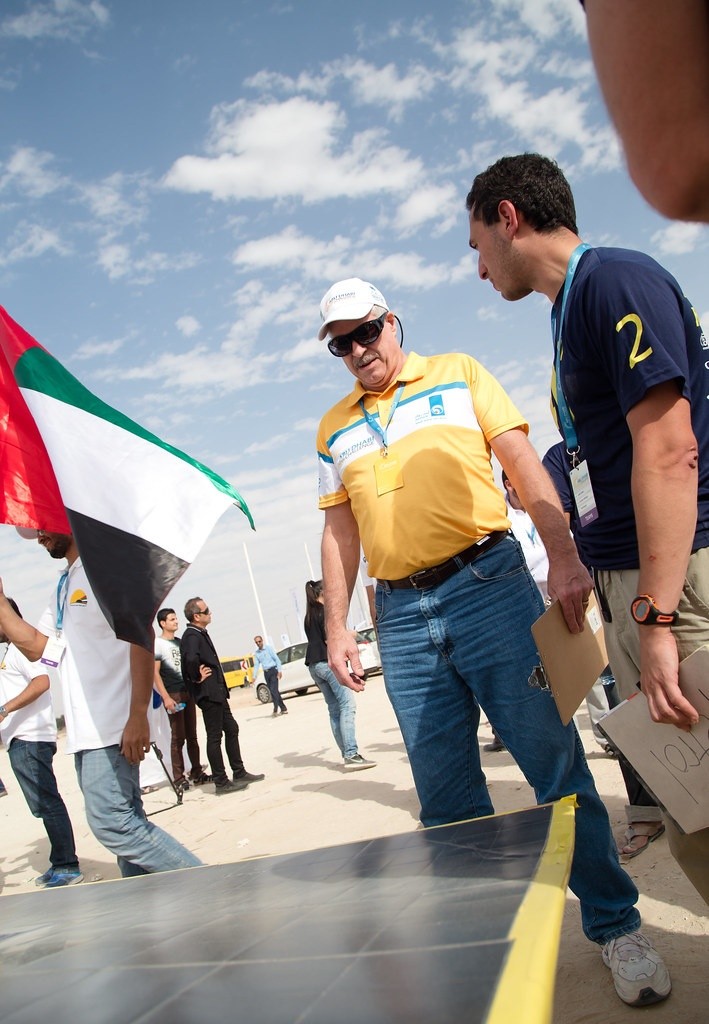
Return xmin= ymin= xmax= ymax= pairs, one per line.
xmin=263 ymin=669 xmax=271 ymax=673
xmin=376 ymin=528 xmax=510 ymax=592
xmin=592 ymin=567 xmax=611 ymax=623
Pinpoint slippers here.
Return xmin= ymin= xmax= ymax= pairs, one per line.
xmin=617 ymin=825 xmax=665 ymax=862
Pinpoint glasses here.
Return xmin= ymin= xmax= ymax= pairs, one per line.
xmin=37 ymin=531 xmax=45 ymax=536
xmin=327 ymin=310 xmax=387 ymax=358
xmin=255 ymin=640 xmax=262 ymax=643
xmin=194 ymin=607 xmax=209 ymax=615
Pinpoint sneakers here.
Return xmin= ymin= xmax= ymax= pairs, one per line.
xmin=599 ymin=925 xmax=672 ymax=1007
xmin=343 ymin=752 xmax=377 ymax=769
xmin=35 ymin=864 xmax=84 ymax=889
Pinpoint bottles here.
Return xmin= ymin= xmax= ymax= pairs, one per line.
xmin=167 ymin=703 xmax=186 ymax=714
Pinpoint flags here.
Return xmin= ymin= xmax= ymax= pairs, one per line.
xmin=0 ymin=306 xmax=259 ymax=656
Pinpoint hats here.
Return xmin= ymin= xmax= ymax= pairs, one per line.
xmin=318 ymin=277 xmax=389 ymax=341
xmin=16 ymin=524 xmax=37 ymax=539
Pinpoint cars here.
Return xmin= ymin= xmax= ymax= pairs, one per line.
xmin=253 ymin=629 xmax=376 ymax=704
xmin=354 ymin=624 xmax=382 ymax=669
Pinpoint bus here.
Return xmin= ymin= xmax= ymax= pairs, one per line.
xmin=219 ymin=653 xmax=261 ymax=690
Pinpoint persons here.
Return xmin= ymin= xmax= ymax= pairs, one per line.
xmin=0 ymin=521 xmax=266 ymax=889
xmin=304 ymin=580 xmax=379 ymax=770
xmin=252 ymin=635 xmax=289 ymax=716
xmin=322 ymin=0 xmax=709 ymax=1024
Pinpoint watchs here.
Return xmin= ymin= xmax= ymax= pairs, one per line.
xmin=630 ymin=593 xmax=680 ymax=626
xmin=0 ymin=706 xmax=9 ymax=717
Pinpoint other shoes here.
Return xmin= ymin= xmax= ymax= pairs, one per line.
xmin=0 ymin=789 xmax=7 ymax=798
xmin=176 ymin=773 xmax=214 ymax=790
xmin=271 ymin=711 xmax=288 ymax=717
xmin=233 ymin=772 xmax=265 ymax=782
xmin=215 ymin=780 xmax=248 ymax=795
xmin=605 ymin=745 xmax=616 ymax=757
xmin=484 ymin=739 xmax=505 ymax=752
